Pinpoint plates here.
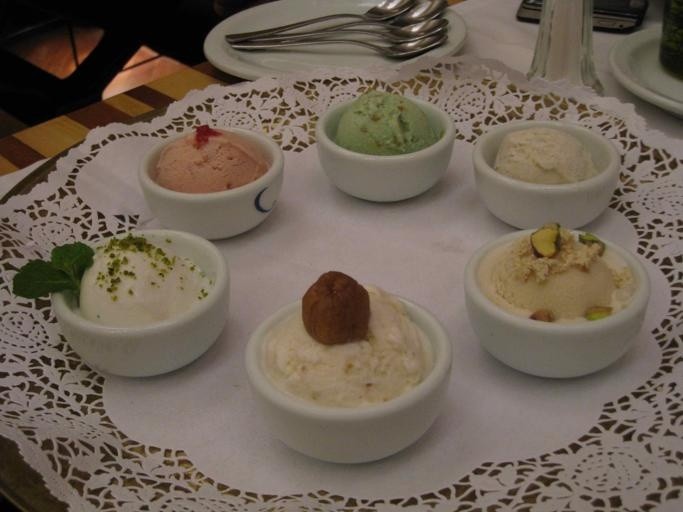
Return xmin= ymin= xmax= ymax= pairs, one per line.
xmin=202 ymin=2 xmax=465 ymax=83
xmin=609 ymin=21 xmax=683 ymax=114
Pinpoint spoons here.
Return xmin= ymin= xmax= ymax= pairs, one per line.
xmin=223 ymin=1 xmax=449 ymax=57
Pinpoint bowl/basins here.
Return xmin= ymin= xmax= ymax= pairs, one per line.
xmin=463 ymin=228 xmax=652 ymax=381
xmin=245 ymin=294 xmax=452 ymax=468
xmin=50 ymin=229 xmax=230 ymax=380
xmin=137 ymin=125 xmax=284 ymax=240
xmin=471 ymin=118 xmax=623 ymax=226
xmin=316 ymin=97 xmax=457 ymax=205
